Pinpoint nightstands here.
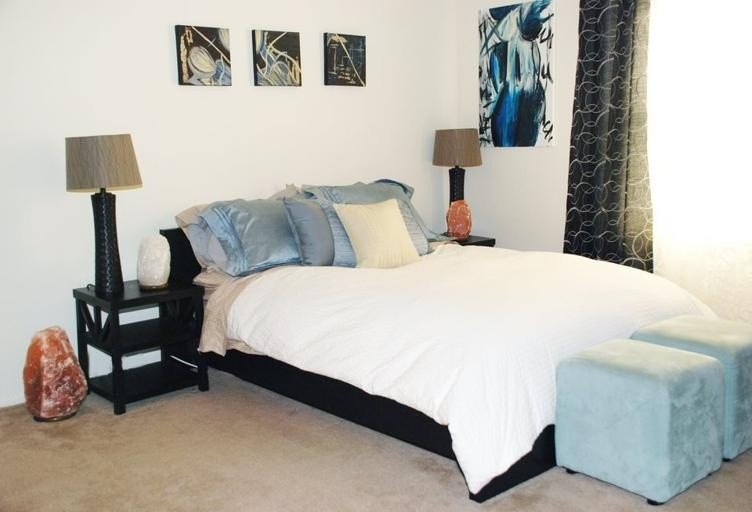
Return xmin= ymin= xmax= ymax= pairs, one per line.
xmin=441 ymin=232 xmax=496 ymax=247
xmin=72 ymin=280 xmax=211 ymax=414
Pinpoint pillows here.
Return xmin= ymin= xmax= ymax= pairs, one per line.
xmin=176 ymin=179 xmax=435 ymax=277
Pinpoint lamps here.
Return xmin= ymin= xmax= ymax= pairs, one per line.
xmin=433 ymin=128 xmax=481 ymax=234
xmin=65 ymin=133 xmax=143 ymax=293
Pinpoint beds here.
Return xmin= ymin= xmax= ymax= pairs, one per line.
xmin=159 ymin=227 xmax=721 ymax=503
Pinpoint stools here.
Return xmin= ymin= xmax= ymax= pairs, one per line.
xmin=555 ymin=338 xmax=723 ymax=506
xmin=629 ymin=314 xmax=752 ymax=462
